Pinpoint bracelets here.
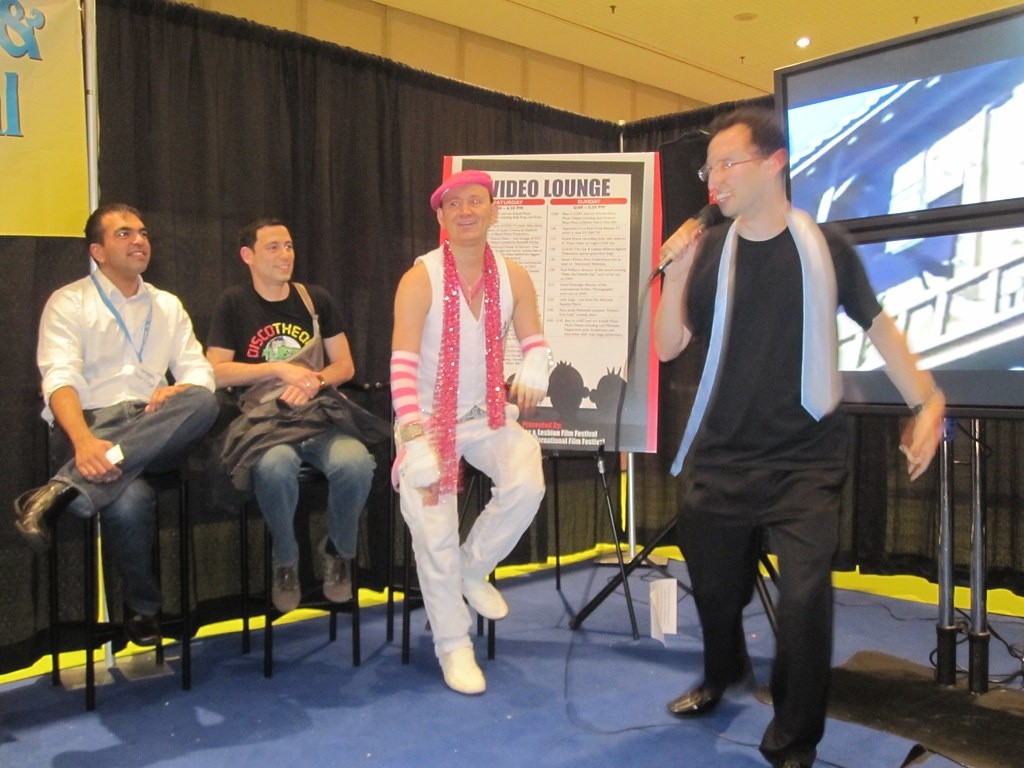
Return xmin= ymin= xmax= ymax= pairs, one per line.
xmin=399 ymin=422 xmax=423 ymax=441
xmin=318 ymin=377 xmax=324 ymax=389
xmin=911 ymin=391 xmax=941 ymax=415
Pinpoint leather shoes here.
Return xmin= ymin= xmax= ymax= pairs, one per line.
xmin=11 ymin=481 xmax=76 ymax=545
xmin=123 ymin=608 xmax=163 ymax=649
xmin=666 ymin=670 xmax=755 ymax=717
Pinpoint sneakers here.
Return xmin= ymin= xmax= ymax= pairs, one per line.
xmin=440 ymin=640 xmax=486 ymax=695
xmin=273 ymin=544 xmax=301 ymax=612
xmin=318 ymin=534 xmax=353 ymax=604
xmin=461 ymin=579 xmax=509 ymax=621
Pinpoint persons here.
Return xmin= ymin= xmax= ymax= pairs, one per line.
xmin=205 ymin=218 xmax=378 ymax=612
xmin=655 ymin=111 xmax=946 ymax=768
xmin=14 ymin=203 xmax=221 ymax=645
xmin=389 ymin=169 xmax=549 ymax=694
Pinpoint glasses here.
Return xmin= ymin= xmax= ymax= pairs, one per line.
xmin=698 ymin=155 xmax=770 ymax=182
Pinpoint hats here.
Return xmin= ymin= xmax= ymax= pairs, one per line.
xmin=430 ymin=169 xmax=494 ymax=211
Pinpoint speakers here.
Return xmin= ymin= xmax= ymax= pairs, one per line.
xmin=661 ymin=131 xmax=713 ymax=241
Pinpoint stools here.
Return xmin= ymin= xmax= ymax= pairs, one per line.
xmin=386 ymin=458 xmax=499 ymax=665
xmin=26 ymin=395 xmax=195 ymax=707
xmin=239 ymin=463 xmax=362 ymax=677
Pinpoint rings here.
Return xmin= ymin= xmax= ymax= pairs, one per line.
xmin=307 ymin=383 xmax=310 ymax=387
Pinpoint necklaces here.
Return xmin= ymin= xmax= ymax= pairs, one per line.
xmin=461 ymin=275 xmax=482 ymax=306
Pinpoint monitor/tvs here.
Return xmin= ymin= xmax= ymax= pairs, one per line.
xmin=772 ymin=0 xmax=1024 ymax=424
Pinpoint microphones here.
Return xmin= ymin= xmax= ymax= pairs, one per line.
xmin=649 ymin=203 xmax=725 ymax=282
